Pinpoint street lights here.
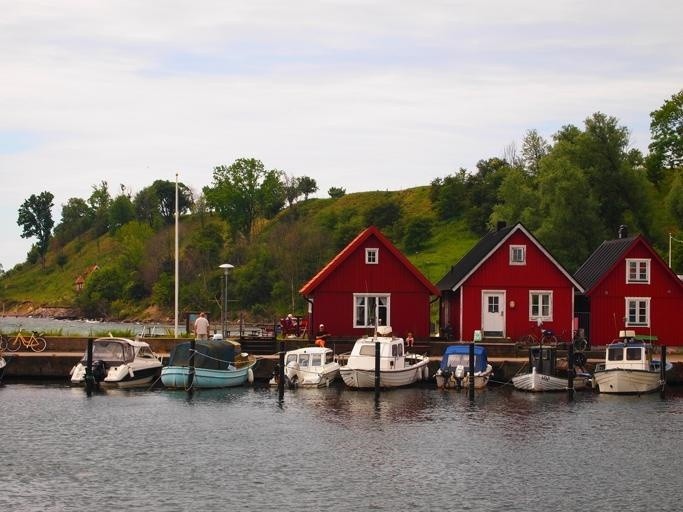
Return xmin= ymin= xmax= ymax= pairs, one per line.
xmin=217 ymin=263 xmax=235 ymax=337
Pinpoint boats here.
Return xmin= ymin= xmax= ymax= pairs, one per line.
xmin=593 ymin=316 xmax=674 ymax=393
xmin=268 ymin=346 xmax=340 ymax=391
xmin=511 ymin=343 xmax=590 ymax=393
xmin=160 ymin=338 xmax=258 ymax=389
xmin=435 ymin=344 xmax=493 ymax=391
xmin=68 ymin=335 xmax=164 ymax=388
xmin=338 ymin=335 xmax=431 ymax=390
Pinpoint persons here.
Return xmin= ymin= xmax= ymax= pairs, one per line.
xmin=403 ymin=332 xmax=414 ymax=353
xmin=286 ymin=313 xmax=301 ymax=337
xmin=313 ymin=323 xmax=331 ymax=348
xmin=438 ymin=320 xmax=453 ymax=341
xmin=193 ymin=311 xmax=209 ymax=342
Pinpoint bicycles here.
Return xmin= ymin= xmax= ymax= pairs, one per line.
xmin=5 ymin=322 xmax=47 ymax=351
